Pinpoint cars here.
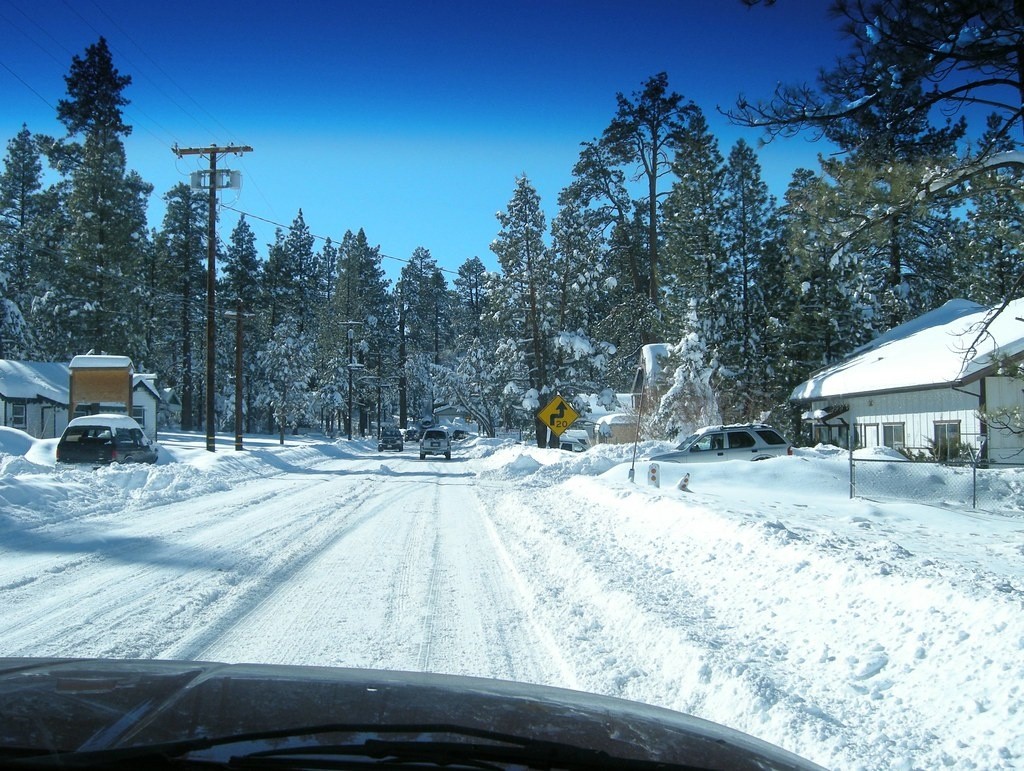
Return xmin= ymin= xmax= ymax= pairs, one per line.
xmin=547 ymin=428 xmax=592 ymax=454
xmin=55 ymin=414 xmax=159 ymax=468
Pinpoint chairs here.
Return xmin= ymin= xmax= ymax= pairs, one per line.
xmin=711 ymin=439 xmax=723 ymax=450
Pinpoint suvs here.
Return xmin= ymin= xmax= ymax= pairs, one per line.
xmin=377 ymin=428 xmax=404 ymax=453
xmin=643 ymin=423 xmax=795 ymax=461
xmin=420 ymin=428 xmax=452 ymax=459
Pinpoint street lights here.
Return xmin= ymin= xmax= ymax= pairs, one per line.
xmin=337 ymin=319 xmax=364 ymax=439
